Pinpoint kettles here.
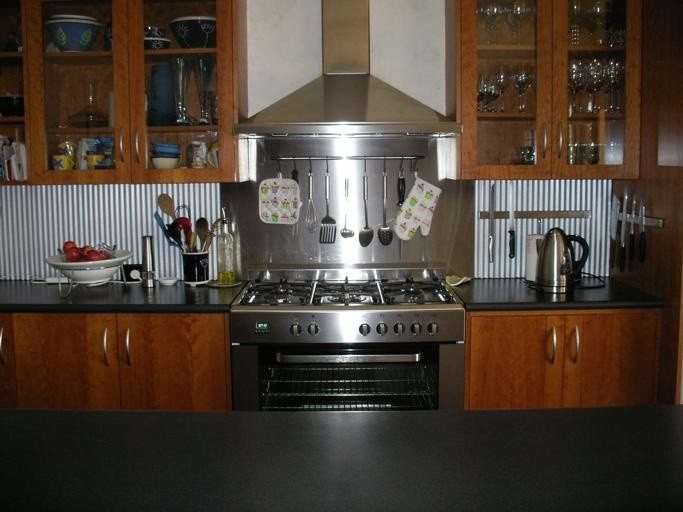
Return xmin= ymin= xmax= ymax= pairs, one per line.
xmin=536 ymin=227 xmax=589 ymax=294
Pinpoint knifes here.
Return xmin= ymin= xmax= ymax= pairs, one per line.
xmin=508 ymin=180 xmax=518 ymax=258
xmin=608 ymin=183 xmax=646 ymax=272
xmin=490 ymin=182 xmax=497 ymax=263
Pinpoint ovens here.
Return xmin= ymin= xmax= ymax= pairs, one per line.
xmin=230 ymin=342 xmax=465 ymax=412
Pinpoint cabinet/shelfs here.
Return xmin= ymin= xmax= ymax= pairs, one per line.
xmin=0 ymin=311 xmax=231 ymax=411
xmin=445 ymin=0 xmax=640 ymax=180
xmin=28 ymin=0 xmax=247 ymax=186
xmin=465 ymin=309 xmax=663 ymax=409
xmin=0 ymin=0 xmax=36 ymax=186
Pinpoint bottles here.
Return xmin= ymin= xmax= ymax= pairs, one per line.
xmin=216 ymin=218 xmax=237 ymax=284
xmin=140 ymin=234 xmax=155 ymax=289
xmin=149 ymin=63 xmax=178 ymax=126
xmin=67 ymin=80 xmax=110 ymax=127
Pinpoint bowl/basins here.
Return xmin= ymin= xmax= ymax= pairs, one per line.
xmin=45 ymin=248 xmax=133 ymax=287
xmin=150 ymin=142 xmax=180 ymax=170
xmin=43 ymin=15 xmax=102 ymax=52
xmin=144 ymin=26 xmax=172 ymax=49
xmin=170 ymin=16 xmax=217 ymax=49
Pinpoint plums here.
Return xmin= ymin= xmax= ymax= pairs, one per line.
xmin=63 ymin=241 xmax=111 ymax=262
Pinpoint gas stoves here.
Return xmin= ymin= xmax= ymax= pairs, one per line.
xmin=230 ymin=276 xmax=466 ymax=341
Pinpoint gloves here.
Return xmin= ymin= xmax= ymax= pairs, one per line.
xmin=395 ymin=178 xmax=442 ymax=241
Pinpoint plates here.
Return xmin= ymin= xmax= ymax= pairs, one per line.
xmin=207 ymin=280 xmax=242 ymax=288
xmin=158 ymin=278 xmax=179 ymax=285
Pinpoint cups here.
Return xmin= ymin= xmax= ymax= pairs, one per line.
xmin=182 ymin=252 xmax=211 ymax=288
xmin=207 ymin=148 xmax=220 ymax=169
xmin=171 ymin=55 xmax=216 ymax=126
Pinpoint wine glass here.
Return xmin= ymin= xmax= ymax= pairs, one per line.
xmin=478 ymin=0 xmax=625 ymax=164
